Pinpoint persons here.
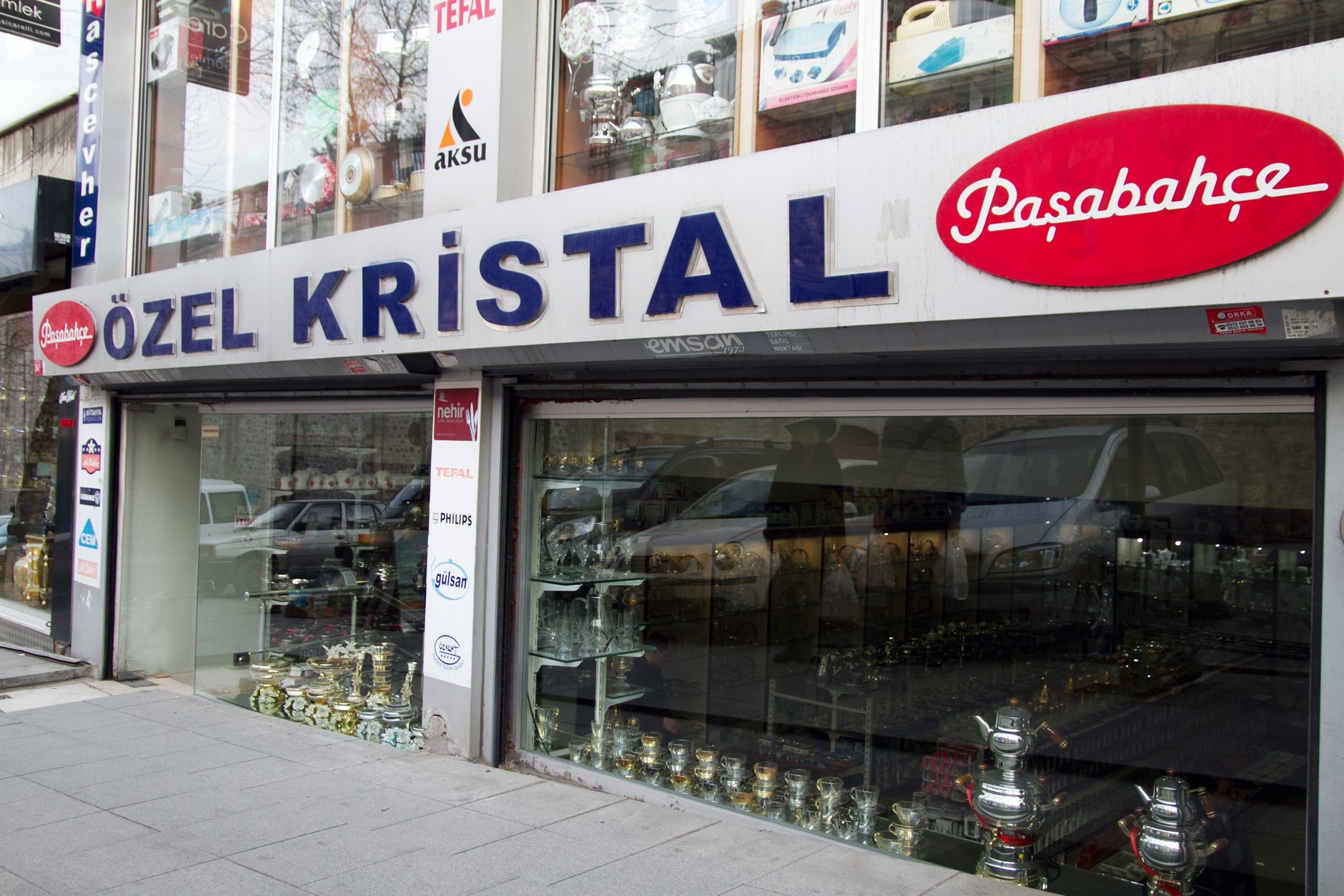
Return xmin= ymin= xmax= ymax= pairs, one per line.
xmin=765 ymin=416 xmax=967 ymax=666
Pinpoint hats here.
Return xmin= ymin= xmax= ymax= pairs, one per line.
xmin=784 ymin=418 xmax=840 ymax=445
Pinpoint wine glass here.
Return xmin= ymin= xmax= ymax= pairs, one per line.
xmin=539 ymin=515 xmax=638 ymax=581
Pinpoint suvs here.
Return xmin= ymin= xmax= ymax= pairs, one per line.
xmin=197 ymin=421 xmax=1234 ymax=673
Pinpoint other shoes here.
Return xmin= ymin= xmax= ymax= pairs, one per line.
xmin=773 ymin=648 xmax=805 ymax=665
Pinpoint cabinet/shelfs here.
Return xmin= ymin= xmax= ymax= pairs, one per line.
xmin=1114 ymin=530 xmax=1312 ymax=642
xmin=530 ymin=475 xmax=660 ymax=760
xmin=767 ymin=638 xmax=1295 ymax=859
xmin=645 ymin=512 xmax=1093 ymax=749
xmin=266 ymin=486 xmax=429 ymax=653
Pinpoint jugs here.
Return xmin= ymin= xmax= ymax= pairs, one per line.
xmin=971 ymin=698 xmax=1068 ymax=772
xmin=1134 ymin=767 xmax=1217 ymax=827
xmin=1156 ymin=548 xmax=1177 ymax=564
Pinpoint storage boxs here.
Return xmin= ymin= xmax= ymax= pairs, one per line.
xmin=1153 ymin=1 xmax=1343 ymax=45
xmin=147 ymin=17 xmax=204 ymax=92
xmin=758 ymin=0 xmax=858 ymax=123
xmin=888 ymin=13 xmax=1014 ymax=98
xmin=1041 ymin=0 xmax=1178 ymax=75
xmin=148 ymin=191 xmax=191 ymax=224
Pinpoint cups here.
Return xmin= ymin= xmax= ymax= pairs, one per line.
xmin=543 ymin=452 xmax=648 ymax=477
xmin=568 ymin=720 xmax=930 ymax=855
xmin=537 ymin=594 xmax=641 ymax=660
xmin=647 ymin=538 xmax=920 ymax=697
xmin=1144 ymin=550 xmax=1156 ymax=570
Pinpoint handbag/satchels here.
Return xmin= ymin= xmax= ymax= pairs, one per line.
xmin=850 ymin=525 xmax=896 ymax=594
xmin=821 ymin=561 xmax=860 ymax=623
xmin=930 ymin=533 xmax=967 ymax=600
xmin=881 ymin=487 xmax=892 ymax=532
